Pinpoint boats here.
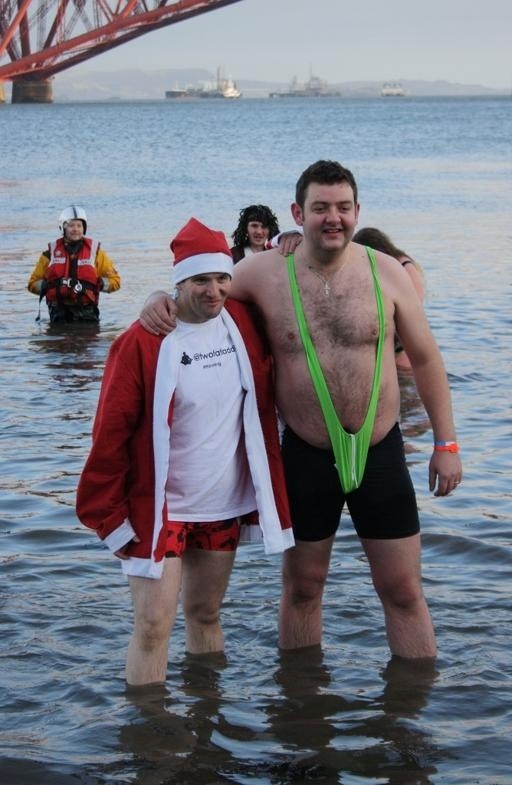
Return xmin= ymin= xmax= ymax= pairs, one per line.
xmin=377 ymin=81 xmax=411 ymax=97
xmin=165 ymin=64 xmax=241 ymax=100
xmin=267 ymin=74 xmax=343 ymax=96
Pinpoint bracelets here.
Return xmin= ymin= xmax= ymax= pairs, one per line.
xmin=433 ymin=441 xmax=459 ymax=453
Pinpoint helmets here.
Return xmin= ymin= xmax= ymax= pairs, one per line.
xmin=56 ymin=205 xmax=89 ymax=235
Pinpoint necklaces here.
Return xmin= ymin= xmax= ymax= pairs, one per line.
xmin=308 ymin=263 xmax=346 ymax=295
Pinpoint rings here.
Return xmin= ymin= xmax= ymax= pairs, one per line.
xmin=454 ymin=482 xmax=460 ymax=484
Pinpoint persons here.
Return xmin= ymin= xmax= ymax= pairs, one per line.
xmin=139 ymin=161 xmax=461 ymax=659
xmin=28 ymin=205 xmax=120 ymax=321
xmin=352 ymin=228 xmax=423 ymax=368
xmin=76 ymin=218 xmax=303 ymax=686
xmin=229 ymin=205 xmax=280 ymax=265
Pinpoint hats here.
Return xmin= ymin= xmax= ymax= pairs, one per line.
xmin=170 ymin=217 xmax=236 ymax=285
